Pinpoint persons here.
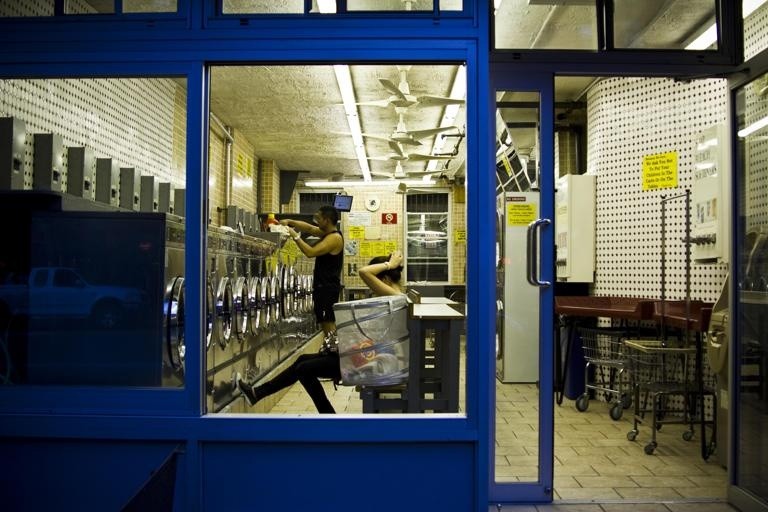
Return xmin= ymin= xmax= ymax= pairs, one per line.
xmin=274 ymin=204 xmax=345 ymax=355
xmin=234 ymin=248 xmax=413 ymax=414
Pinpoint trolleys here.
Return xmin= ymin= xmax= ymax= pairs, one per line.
xmin=622 ymin=339 xmax=722 ymax=455
xmin=572 ymin=323 xmax=686 ymax=422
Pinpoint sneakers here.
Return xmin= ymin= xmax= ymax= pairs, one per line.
xmin=235 ymin=371 xmax=259 ymax=407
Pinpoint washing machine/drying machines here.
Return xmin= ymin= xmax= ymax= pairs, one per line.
xmin=1 ymin=190 xmax=323 ymax=414
xmin=464 ymin=190 xmax=541 ymax=384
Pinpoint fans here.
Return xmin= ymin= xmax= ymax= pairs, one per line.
xmin=331 ymin=1 xmax=468 ymax=198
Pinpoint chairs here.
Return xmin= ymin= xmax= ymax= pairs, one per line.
xmin=352 ymin=290 xmax=422 ymax=413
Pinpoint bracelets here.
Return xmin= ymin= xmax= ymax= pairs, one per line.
xmin=293 ymin=231 xmax=302 ymax=241
xmin=384 ymin=261 xmax=390 ymax=271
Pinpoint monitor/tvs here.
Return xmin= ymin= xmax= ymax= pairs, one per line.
xmin=332 ymin=195 xmax=353 ymax=213
